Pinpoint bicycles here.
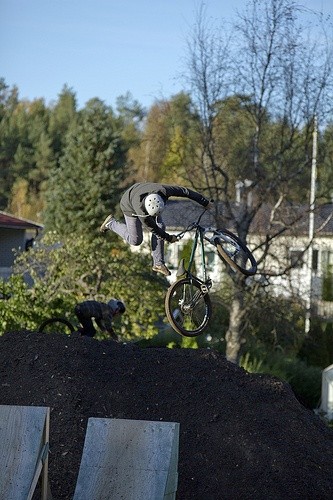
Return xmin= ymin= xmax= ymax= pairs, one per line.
xmin=38 ymin=317 xmax=87 ymax=339
xmin=163 ymin=199 xmax=259 ymax=337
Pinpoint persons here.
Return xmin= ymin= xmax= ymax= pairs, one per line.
xmin=100 ymin=183 xmax=212 ymax=276
xmin=74 ymin=299 xmax=126 ymax=339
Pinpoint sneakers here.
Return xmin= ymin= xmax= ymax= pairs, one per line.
xmin=100 ymin=214 xmax=115 ymax=233
xmin=152 ymin=264 xmax=171 ymax=276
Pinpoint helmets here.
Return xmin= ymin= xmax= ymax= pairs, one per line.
xmin=108 ymin=298 xmax=126 ymax=315
xmin=145 ymin=193 xmax=164 ymax=216
